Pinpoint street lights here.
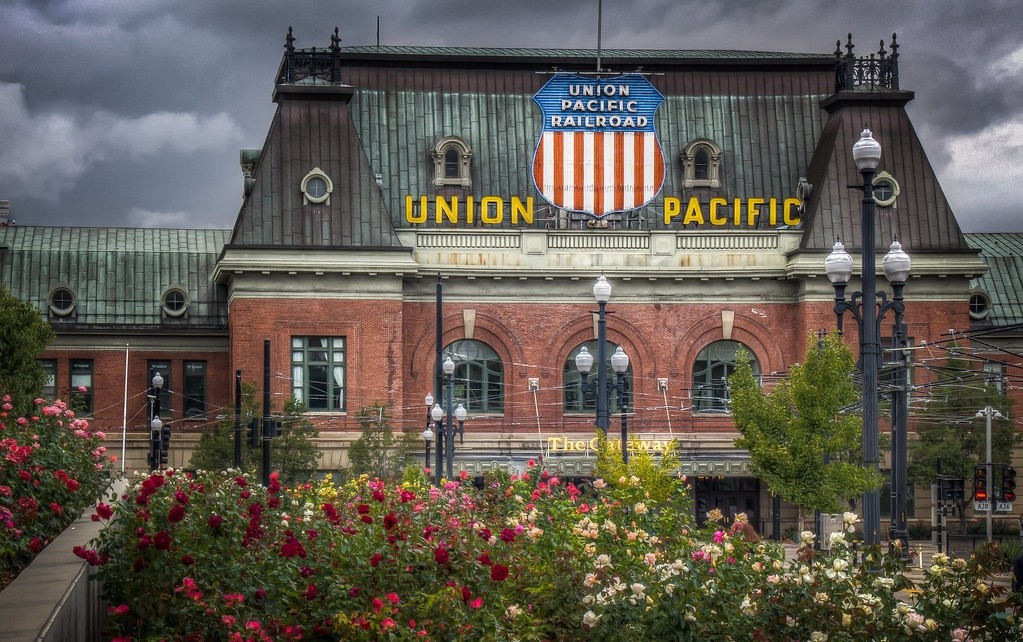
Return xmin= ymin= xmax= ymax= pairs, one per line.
xmin=425 ymin=392 xmax=433 ymax=478
xmin=574 ymin=276 xmax=631 ymax=456
xmin=822 ymin=121 xmax=916 ymax=573
xmin=149 ymin=370 xmax=164 ymax=473
xmin=430 ymin=356 xmax=471 ymax=482
